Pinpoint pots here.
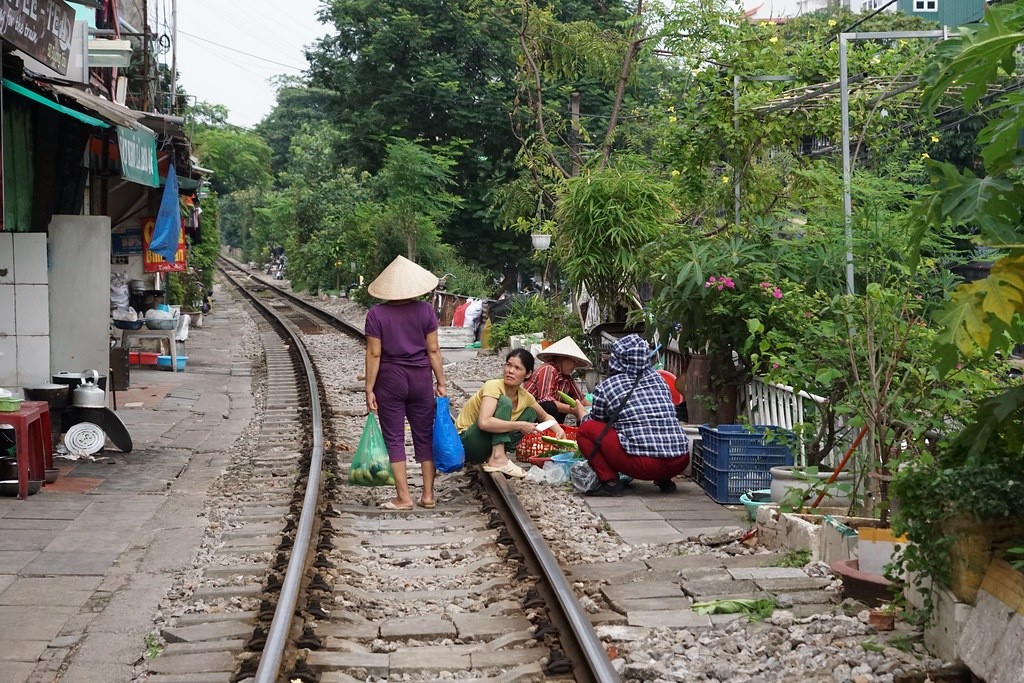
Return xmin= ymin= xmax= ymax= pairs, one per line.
xmin=112 ymin=319 xmax=142 ymax=330
xmin=23 ymin=383 xmax=69 ymax=408
xmin=53 ymin=371 xmax=108 ymax=403
xmin=144 ymin=289 xmax=166 ymax=297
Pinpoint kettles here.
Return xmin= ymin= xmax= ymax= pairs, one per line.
xmin=73 ymin=369 xmax=106 ymax=408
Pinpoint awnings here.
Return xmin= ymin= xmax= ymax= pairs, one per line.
xmin=2 ymin=78 xmax=159 ymax=188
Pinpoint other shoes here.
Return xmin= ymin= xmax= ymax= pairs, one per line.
xmin=653 ymin=479 xmax=677 ymax=493
xmin=585 ymin=481 xmax=624 ymax=496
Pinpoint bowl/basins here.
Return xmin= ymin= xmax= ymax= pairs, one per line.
xmin=45 ymin=467 xmax=60 ymax=483
xmin=833 ymin=559 xmax=902 ymax=607
xmin=129 ymin=353 xmax=139 ymax=364
xmin=146 ymin=318 xmax=178 ymax=329
xmin=739 ymin=489 xmax=771 ymax=521
xmin=657 ymin=370 xmax=683 ymax=405
xmin=140 ymin=352 xmax=161 ymax=364
xmin=158 ymin=356 xmax=188 ymax=369
xmin=0 ymin=480 xmax=44 ymax=496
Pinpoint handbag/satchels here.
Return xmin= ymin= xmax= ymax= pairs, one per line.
xmin=348 ymin=412 xmax=395 ymax=486
xmin=528 ymin=452 xmax=600 ymax=494
xmin=451 ymin=296 xmax=483 ymax=327
xmin=431 ymin=395 xmax=465 ymax=473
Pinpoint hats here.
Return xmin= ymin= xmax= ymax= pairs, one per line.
xmin=608 ymin=333 xmax=652 ymax=373
xmin=537 ymin=335 xmax=593 ymax=369
xmin=367 ymin=255 xmax=438 ymax=300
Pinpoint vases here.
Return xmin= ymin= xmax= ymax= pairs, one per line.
xmin=768 ymin=466 xmax=858 ymax=509
xmin=681 ymin=354 xmax=726 ymax=423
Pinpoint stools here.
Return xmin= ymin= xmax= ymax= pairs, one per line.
xmin=0 ymin=400 xmax=50 ymax=499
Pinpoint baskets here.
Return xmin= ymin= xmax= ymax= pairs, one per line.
xmin=618 ymin=473 xmax=634 ymax=485
xmin=529 ymin=453 xmax=552 ymax=468
xmin=515 ymin=421 xmax=580 ymax=462
xmin=690 ymin=423 xmax=798 ymax=504
xmin=0 ymin=398 xmax=25 ymax=411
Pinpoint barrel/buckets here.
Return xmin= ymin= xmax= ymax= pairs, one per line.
xmin=130 ymin=279 xmax=145 ymax=295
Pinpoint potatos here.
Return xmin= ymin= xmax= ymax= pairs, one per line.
xmin=349 ymin=464 xmax=397 ymax=485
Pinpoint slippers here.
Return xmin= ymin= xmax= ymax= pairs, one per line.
xmin=416 ymin=498 xmax=435 ymax=508
xmin=377 ymin=501 xmax=413 ymax=510
xmin=481 ymin=458 xmax=527 ymax=478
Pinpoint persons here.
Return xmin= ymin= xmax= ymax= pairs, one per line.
xmin=454 ymin=349 xmax=566 ymax=478
xmin=365 ymin=255 xmax=447 ymax=509
xmin=570 ymin=333 xmax=690 ymax=497
xmin=524 ymin=335 xmax=593 ymax=425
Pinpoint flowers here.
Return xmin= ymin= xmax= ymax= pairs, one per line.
xmin=624 ymin=231 xmax=794 ymax=377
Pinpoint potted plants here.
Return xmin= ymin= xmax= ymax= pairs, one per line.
xmin=510 ymin=215 xmax=559 ymax=250
xmin=181 ymin=283 xmax=202 ymax=326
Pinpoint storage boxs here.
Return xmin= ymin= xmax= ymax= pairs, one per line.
xmin=682 ymin=423 xmax=1024 ymax=682
xmin=509 ymin=332 xmax=546 ymax=370
xmin=438 ymin=326 xmax=473 ymax=349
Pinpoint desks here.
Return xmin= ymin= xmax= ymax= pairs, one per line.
xmin=123 ymin=325 xmax=178 ymax=373
